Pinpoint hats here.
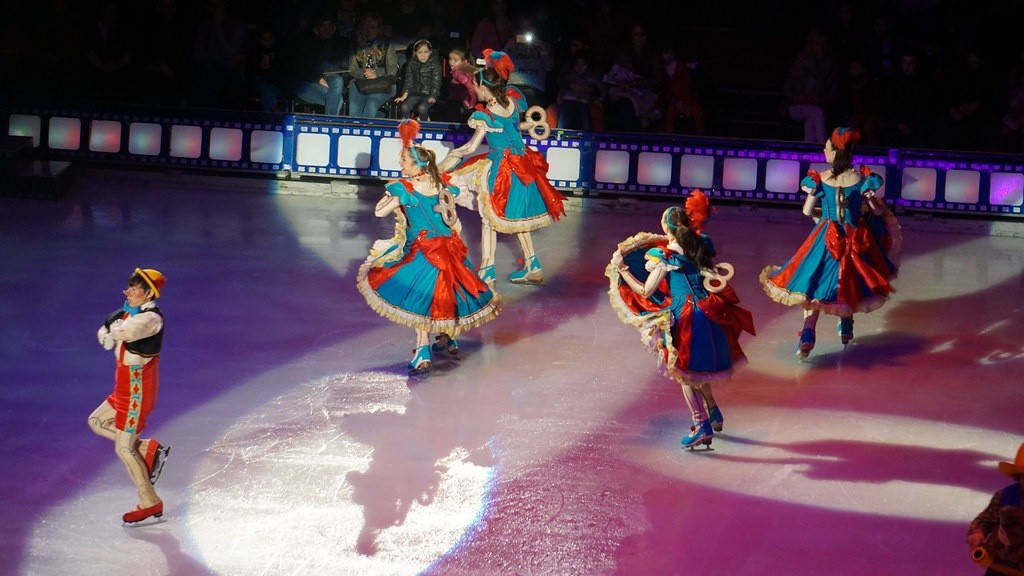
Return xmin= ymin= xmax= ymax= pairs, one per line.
xmin=135 ymin=268 xmax=167 ymax=298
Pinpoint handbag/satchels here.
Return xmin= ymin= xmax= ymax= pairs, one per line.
xmin=355 ymin=75 xmax=399 ymax=94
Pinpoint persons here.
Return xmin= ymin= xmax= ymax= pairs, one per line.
xmin=604 ymin=188 xmax=755 ymax=453
xmin=0 ymin=1 xmax=1023 ymax=153
xmin=87 ymin=268 xmax=167 ymax=524
xmin=758 ymin=126 xmax=902 ymax=363
xmin=965 ymin=443 xmax=1023 ymax=576
xmin=355 ymin=118 xmax=504 ymax=372
xmin=447 ymin=48 xmax=569 ymax=289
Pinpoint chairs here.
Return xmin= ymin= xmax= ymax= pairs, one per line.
xmin=288 ymin=62 xmax=451 ymax=122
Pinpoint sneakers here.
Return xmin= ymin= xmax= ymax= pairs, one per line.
xmin=681 ymin=419 xmax=715 ymax=452
xmin=145 ymin=439 xmax=171 ymax=484
xmin=121 ymin=500 xmax=167 ymax=528
xmin=795 ymin=328 xmax=815 ymax=362
xmin=508 ymin=255 xmax=546 ymax=287
xmin=709 ymin=405 xmax=723 ymax=431
xmin=478 ymin=264 xmax=496 ymax=290
xmin=432 ymin=338 xmax=460 ymax=360
xmin=408 ymin=345 xmax=431 ymax=377
xmin=837 ymin=318 xmax=854 ymax=350
